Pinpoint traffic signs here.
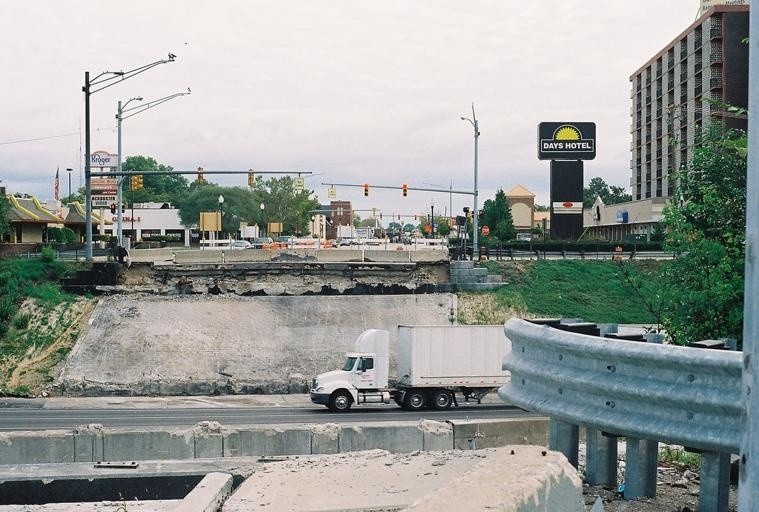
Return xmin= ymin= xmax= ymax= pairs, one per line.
xmin=328 ymin=188 xmax=337 ymax=198
xmin=293 ymin=177 xmax=304 ymax=191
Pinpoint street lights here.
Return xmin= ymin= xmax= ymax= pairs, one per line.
xmin=218 ymin=193 xmax=225 ymax=247
xmin=117 ymin=86 xmax=195 ymax=244
xmin=79 ymin=49 xmax=178 ymax=264
xmin=259 ymin=202 xmax=266 ymax=236
xmin=458 ymin=114 xmax=480 ymax=254
xmin=66 ymin=167 xmax=74 ymax=204
xmin=430 ymin=205 xmax=435 ymax=238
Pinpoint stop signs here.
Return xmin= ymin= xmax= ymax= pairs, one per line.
xmin=481 ymin=225 xmax=490 ymax=236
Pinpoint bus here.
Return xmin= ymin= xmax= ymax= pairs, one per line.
xmin=415 ymin=236 xmax=448 ymax=250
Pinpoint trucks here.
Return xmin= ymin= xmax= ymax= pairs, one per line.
xmin=303 ymin=321 xmax=603 ymax=414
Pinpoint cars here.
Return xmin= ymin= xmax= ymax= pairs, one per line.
xmin=224 ymin=230 xmax=415 ymax=250
xmin=449 ymin=244 xmax=475 ymax=260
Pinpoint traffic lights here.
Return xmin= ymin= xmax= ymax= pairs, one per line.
xmin=364 ymin=183 xmax=368 ymax=196
xmin=137 ymin=174 xmax=144 ymax=191
xmin=403 ymin=183 xmax=408 ymax=196
xmin=130 ymin=175 xmax=138 ymax=192
xmin=111 ymin=203 xmax=115 ymax=215
